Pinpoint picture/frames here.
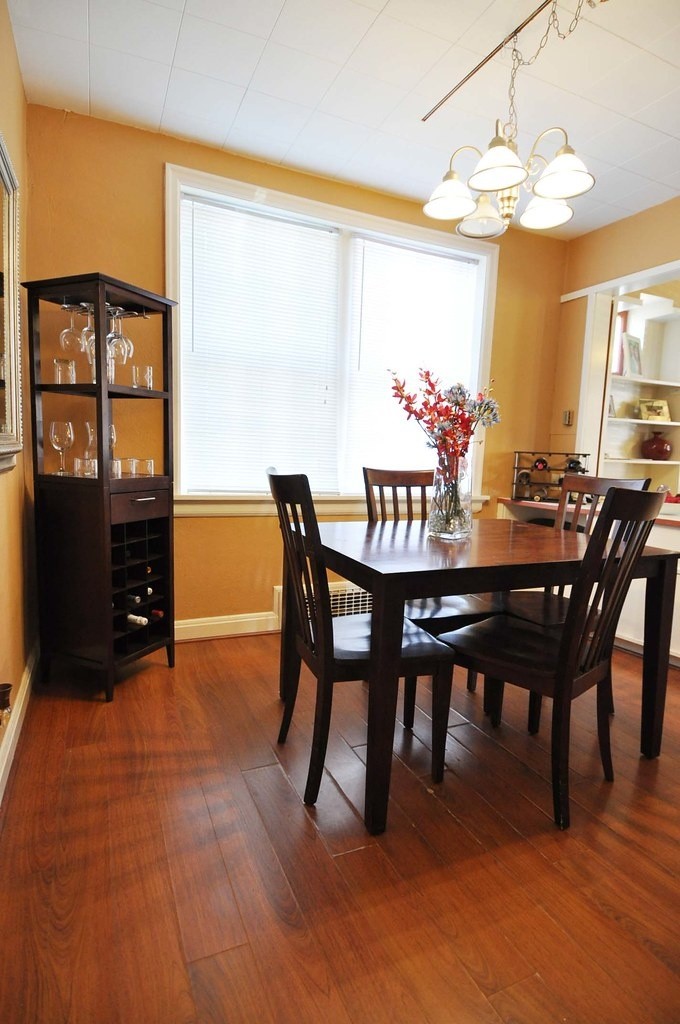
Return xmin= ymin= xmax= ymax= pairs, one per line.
xmin=622 ymin=332 xmax=643 ymax=378
xmin=638 ymin=398 xmax=672 ymax=422
xmin=1 ymin=136 xmax=25 ymax=472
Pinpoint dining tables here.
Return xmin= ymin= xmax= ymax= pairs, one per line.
xmin=280 ymin=517 xmax=679 ymax=835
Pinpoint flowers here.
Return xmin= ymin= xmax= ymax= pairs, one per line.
xmin=387 ymin=366 xmax=502 ymax=531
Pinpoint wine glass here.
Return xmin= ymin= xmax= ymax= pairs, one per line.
xmin=74 ymin=420 xmax=117 ymax=460
xmin=80 ymin=302 xmax=134 ymax=365
xmin=60 ymin=304 xmax=85 ymax=352
xmin=50 ymin=421 xmax=75 ymax=476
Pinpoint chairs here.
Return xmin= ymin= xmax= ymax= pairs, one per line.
xmin=264 ymin=466 xmax=458 ymax=807
xmin=434 ymin=487 xmax=669 ymax=831
xmin=468 ymin=472 xmax=652 ymax=642
xmin=362 ymin=466 xmax=501 ymax=693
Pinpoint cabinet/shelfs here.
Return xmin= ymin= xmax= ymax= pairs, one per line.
xmin=19 ymin=271 xmax=179 ymax=702
xmin=511 ymin=450 xmax=590 ymax=504
xmin=602 ymin=297 xmax=679 ymax=516
xmin=494 ymin=497 xmax=680 ymax=672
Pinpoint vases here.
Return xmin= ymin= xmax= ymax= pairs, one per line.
xmin=427 ymin=455 xmax=475 ymax=541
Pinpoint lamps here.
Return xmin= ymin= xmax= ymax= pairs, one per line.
xmin=420 ymin=1 xmax=608 ymax=239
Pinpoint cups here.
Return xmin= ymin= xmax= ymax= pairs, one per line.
xmin=120 ymin=458 xmax=136 ymax=478
xmin=73 ymin=457 xmax=122 ymax=480
xmin=132 ymin=365 xmax=153 ymax=391
xmin=90 ymin=358 xmax=115 ymax=384
xmin=53 ymin=358 xmax=76 ymax=384
xmin=136 ymin=459 xmax=154 ymax=478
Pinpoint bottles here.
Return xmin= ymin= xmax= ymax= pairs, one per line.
xmin=563 ymin=457 xmax=582 ymax=472
xmin=531 ymin=488 xmax=548 ymax=502
xmin=517 ymin=469 xmax=532 ymax=484
xmin=128 ymin=567 xmax=164 ymax=625
xmin=534 ymin=456 xmax=548 ymax=471
